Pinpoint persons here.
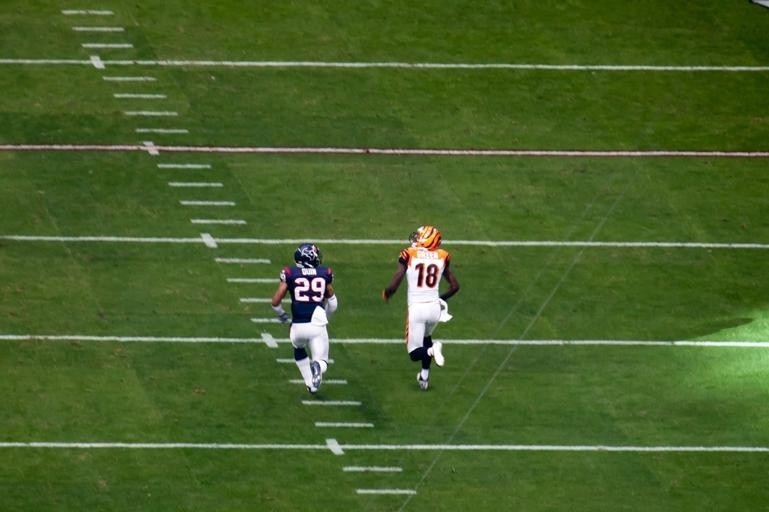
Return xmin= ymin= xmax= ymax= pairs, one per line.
xmin=272 ymin=243 xmax=338 ymax=391
xmin=384 ymin=226 xmax=459 ymax=390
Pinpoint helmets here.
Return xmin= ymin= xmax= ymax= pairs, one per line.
xmin=416 ymin=225 xmax=442 ymax=250
xmin=295 ymin=244 xmax=321 ymax=263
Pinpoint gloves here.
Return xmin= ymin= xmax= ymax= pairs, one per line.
xmin=277 ymin=313 xmax=291 ymax=325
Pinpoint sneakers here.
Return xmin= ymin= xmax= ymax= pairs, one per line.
xmin=432 ymin=341 xmax=445 ymax=367
xmin=306 ymin=360 xmax=321 ymax=394
xmin=416 ymin=372 xmax=429 ymax=389
